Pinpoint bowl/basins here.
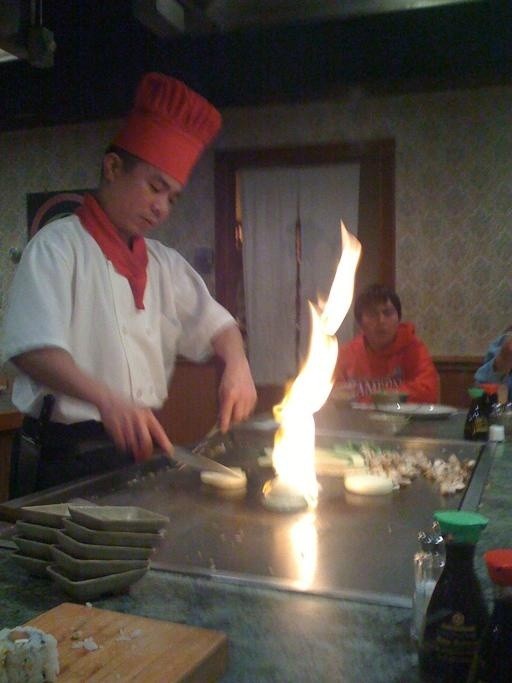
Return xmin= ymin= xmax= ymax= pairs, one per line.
xmin=372 ymin=392 xmax=409 ymax=408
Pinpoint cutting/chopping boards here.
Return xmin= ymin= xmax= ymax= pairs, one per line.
xmin=0 ymin=602 xmax=228 ymax=682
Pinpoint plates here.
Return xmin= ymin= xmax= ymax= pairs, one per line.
xmin=377 ymin=402 xmax=456 ymax=419
xmin=9 ymin=503 xmax=169 ymax=600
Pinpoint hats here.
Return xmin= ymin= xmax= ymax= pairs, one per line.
xmin=114 ymin=67 xmax=223 ymax=187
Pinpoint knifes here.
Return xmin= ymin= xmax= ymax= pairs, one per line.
xmin=14 ymin=394 xmax=57 ymax=497
xmin=151 ymin=439 xmax=241 ymax=479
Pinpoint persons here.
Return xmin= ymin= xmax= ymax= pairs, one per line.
xmin=326 ymin=280 xmax=441 ymax=406
xmin=0 ymin=118 xmax=260 ymax=500
xmin=471 ymin=320 xmax=511 ymax=406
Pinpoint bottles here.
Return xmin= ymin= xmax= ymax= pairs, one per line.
xmin=411 ymin=510 xmax=511 ymax=682
xmin=464 ymin=384 xmax=512 ymax=441
